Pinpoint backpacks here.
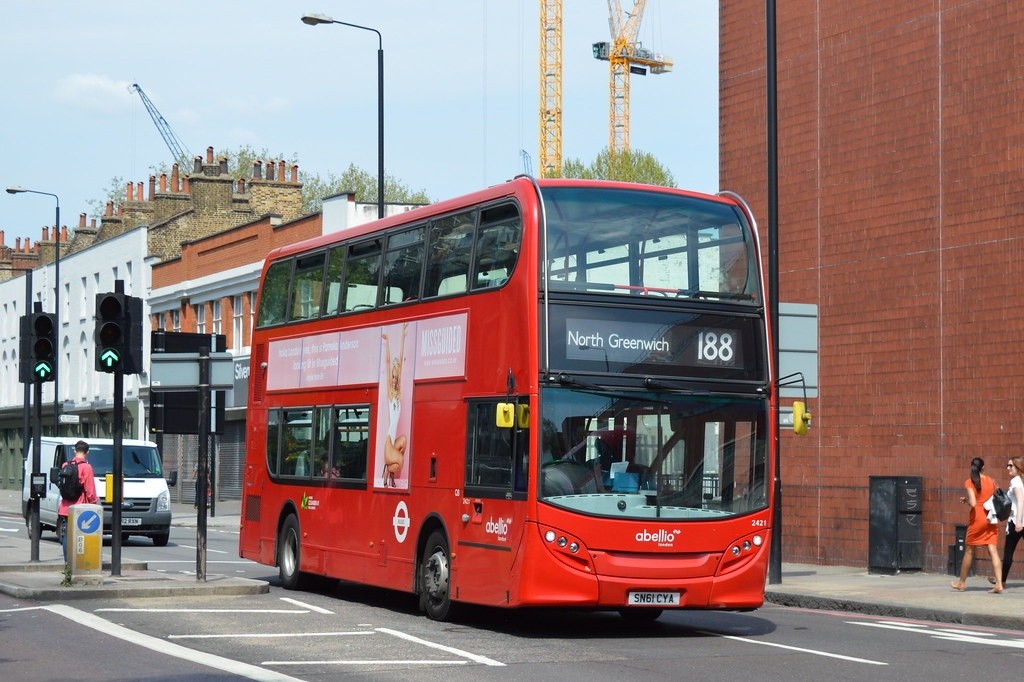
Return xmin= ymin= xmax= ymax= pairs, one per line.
xmin=58 ymin=461 xmax=87 ymax=500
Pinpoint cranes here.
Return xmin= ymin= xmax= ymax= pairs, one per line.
xmin=536 ymin=0 xmax=565 ymax=178
xmin=128 ymin=82 xmax=196 ymax=176
xmin=592 ymin=0 xmax=674 ymax=180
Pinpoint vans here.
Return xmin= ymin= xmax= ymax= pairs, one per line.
xmin=20 ymin=436 xmax=178 ymax=549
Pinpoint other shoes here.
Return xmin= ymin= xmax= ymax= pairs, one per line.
xmin=950 ymin=581 xmax=965 ymax=591
xmin=987 ymin=577 xmax=1005 ymax=588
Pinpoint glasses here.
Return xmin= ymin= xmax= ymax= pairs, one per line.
xmin=1007 ymin=464 xmax=1014 ymax=468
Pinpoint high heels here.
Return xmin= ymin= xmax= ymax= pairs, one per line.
xmin=382 ymin=463 xmax=396 ymax=488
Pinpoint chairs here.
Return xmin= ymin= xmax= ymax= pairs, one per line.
xmin=269 ymin=277 xmax=709 ymax=509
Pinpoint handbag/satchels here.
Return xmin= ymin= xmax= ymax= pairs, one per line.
xmin=993 ymin=488 xmax=1012 ymax=522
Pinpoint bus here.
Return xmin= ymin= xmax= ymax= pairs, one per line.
xmin=236 ymin=173 xmax=811 ymax=623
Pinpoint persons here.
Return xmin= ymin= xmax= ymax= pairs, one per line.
xmin=56 ymin=439 xmax=98 ymax=574
xmin=988 ymin=456 xmax=1024 ymax=586
xmin=379 ymin=320 xmax=409 ymax=489
xmin=950 ymin=458 xmax=1004 ymax=593
xmin=312 ymin=429 xmax=342 ymax=479
xmin=189 ymin=455 xmax=212 ymax=509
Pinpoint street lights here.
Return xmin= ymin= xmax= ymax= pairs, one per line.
xmin=6 ymin=182 xmax=63 ymax=434
xmin=300 ymin=10 xmax=387 ymax=219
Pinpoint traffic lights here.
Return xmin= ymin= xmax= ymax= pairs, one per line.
xmin=94 ymin=293 xmax=126 ymax=374
xmin=31 ymin=312 xmax=59 ymax=382
